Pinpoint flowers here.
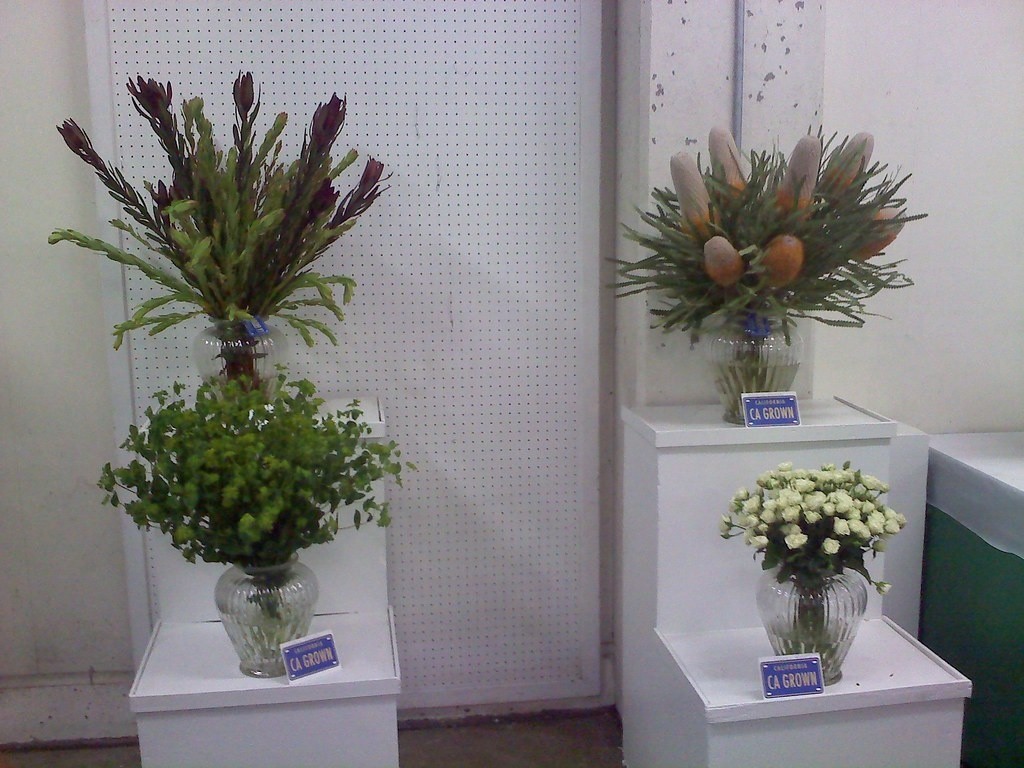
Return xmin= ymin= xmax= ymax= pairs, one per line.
xmin=612 ymin=123 xmax=930 ymax=350
xmin=721 ymin=457 xmax=898 ymax=597
xmin=49 ymin=73 xmax=391 ymax=351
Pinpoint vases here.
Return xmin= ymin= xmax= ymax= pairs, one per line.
xmin=193 ymin=320 xmax=291 ymax=401
xmin=703 ymin=316 xmax=802 ymax=423
xmin=755 ymin=564 xmax=868 ymax=687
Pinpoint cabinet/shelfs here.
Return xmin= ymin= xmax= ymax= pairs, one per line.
xmin=615 ymin=403 xmax=975 ymax=767
xmin=127 ymin=394 xmax=402 ymax=768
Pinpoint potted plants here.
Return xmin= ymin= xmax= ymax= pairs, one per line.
xmin=98 ymin=365 xmax=420 ymax=680
